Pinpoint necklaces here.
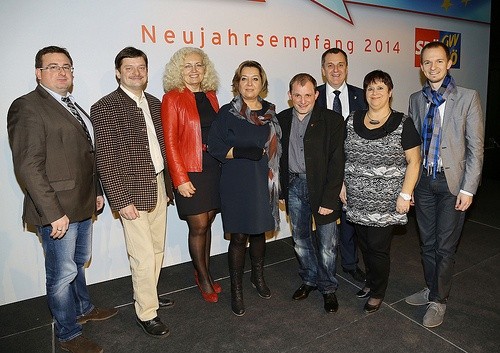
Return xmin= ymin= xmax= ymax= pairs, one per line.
xmin=366 ymin=112 xmax=390 ymax=125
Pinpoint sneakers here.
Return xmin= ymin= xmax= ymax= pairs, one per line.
xmin=405 ymin=288 xmax=432 ymax=305
xmin=422 ymin=300 xmax=446 ymax=327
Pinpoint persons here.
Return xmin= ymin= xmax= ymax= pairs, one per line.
xmin=7 ymin=47 xmax=119 ymax=353
xmin=162 ymin=46 xmax=224 ymax=305
xmin=404 ymin=41 xmax=485 ymax=328
xmin=308 ymin=47 xmax=371 ymax=287
xmin=87 ymin=47 xmax=177 ymax=338
xmin=209 ymin=61 xmax=282 ymax=315
xmin=340 ymin=70 xmax=424 ymax=314
xmin=272 ymin=72 xmax=348 ymax=311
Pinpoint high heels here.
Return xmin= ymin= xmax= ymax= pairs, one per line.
xmin=195 ymin=270 xmax=222 ymax=303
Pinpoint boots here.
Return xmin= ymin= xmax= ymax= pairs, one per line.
xmin=230 ymin=271 xmax=245 ymax=318
xmin=251 ymin=268 xmax=272 ymax=300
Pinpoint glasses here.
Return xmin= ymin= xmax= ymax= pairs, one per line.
xmin=38 ymin=65 xmax=75 ymax=74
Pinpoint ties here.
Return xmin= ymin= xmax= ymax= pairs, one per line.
xmin=61 ymin=96 xmax=93 ymax=149
xmin=332 ymin=91 xmax=343 ymax=114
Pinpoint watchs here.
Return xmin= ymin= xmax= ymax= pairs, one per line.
xmin=399 ymin=192 xmax=413 ymax=202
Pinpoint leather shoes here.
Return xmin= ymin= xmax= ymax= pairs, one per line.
xmin=157 ymin=296 xmax=175 ymax=310
xmin=357 ymin=285 xmax=383 ymax=313
xmin=133 ymin=314 xmax=170 ymax=338
xmin=323 ymin=293 xmax=338 ymax=314
xmin=292 ymin=286 xmax=313 ymax=301
xmin=60 ymin=336 xmax=102 ymax=353
xmin=77 ymin=307 xmax=118 ymax=324
xmin=343 ymin=266 xmax=365 ymax=282
